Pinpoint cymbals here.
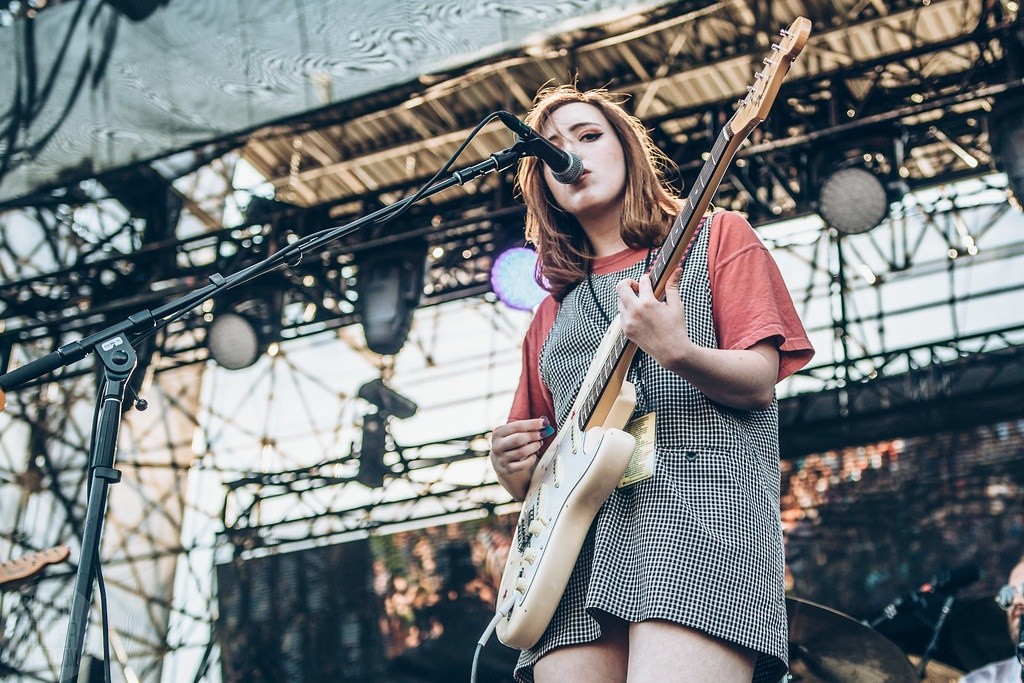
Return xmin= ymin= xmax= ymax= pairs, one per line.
xmin=794 ymin=649 xmax=965 ymax=683
xmin=782 ymin=591 xmax=918 ymax=683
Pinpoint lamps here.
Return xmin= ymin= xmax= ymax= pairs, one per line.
xmin=488 ymin=238 xmax=553 ymax=309
xmin=806 ymin=131 xmax=908 ymax=233
xmin=988 ymin=86 xmax=1024 ymax=208
xmin=353 ymin=237 xmax=428 ymax=354
xmin=207 ymin=292 xmax=282 ymax=369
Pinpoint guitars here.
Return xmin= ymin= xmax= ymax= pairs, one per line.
xmin=493 ymin=15 xmax=813 ymax=651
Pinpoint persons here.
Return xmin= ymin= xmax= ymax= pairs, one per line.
xmin=958 ymin=556 xmax=1024 ymax=683
xmin=490 ymin=85 xmax=814 ymax=683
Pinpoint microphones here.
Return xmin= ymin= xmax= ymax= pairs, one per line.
xmin=498 ymin=110 xmax=584 ymax=184
xmin=887 ymin=560 xmax=981 ymax=619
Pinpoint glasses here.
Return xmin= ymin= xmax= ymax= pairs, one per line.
xmin=994 ymin=583 xmax=1024 ymax=611
